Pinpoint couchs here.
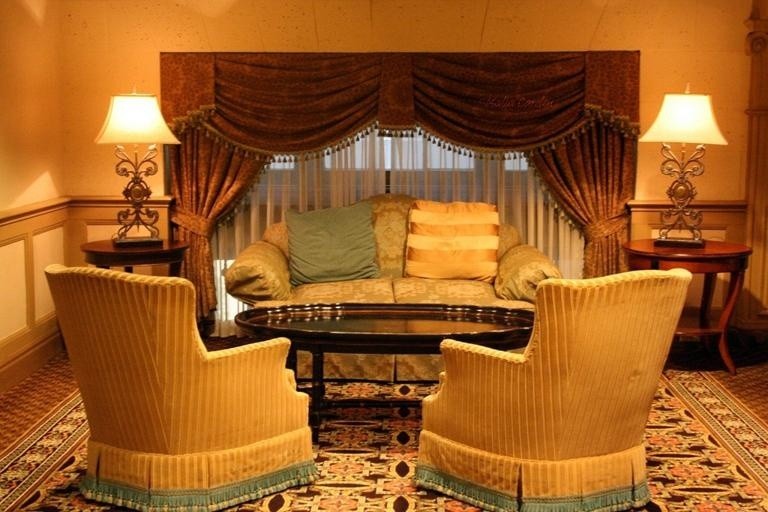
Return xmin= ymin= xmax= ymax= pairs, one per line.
xmin=225 ymin=193 xmax=563 ymax=382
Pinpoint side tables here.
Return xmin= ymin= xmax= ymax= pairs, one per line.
xmin=81 ymin=239 xmax=190 ymax=277
xmin=621 ymin=238 xmax=754 ymax=374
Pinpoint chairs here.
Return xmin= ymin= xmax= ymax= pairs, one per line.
xmin=415 ymin=266 xmax=694 ymax=512
xmin=45 ymin=260 xmax=317 ymax=512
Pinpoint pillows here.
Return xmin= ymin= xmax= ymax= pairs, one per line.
xmin=403 ymin=199 xmax=500 ymax=282
xmin=283 ymin=200 xmax=382 ymax=287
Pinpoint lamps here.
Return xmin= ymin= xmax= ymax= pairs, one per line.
xmin=639 ymin=83 xmax=729 ymax=247
xmin=94 ymin=90 xmax=183 ymax=245
